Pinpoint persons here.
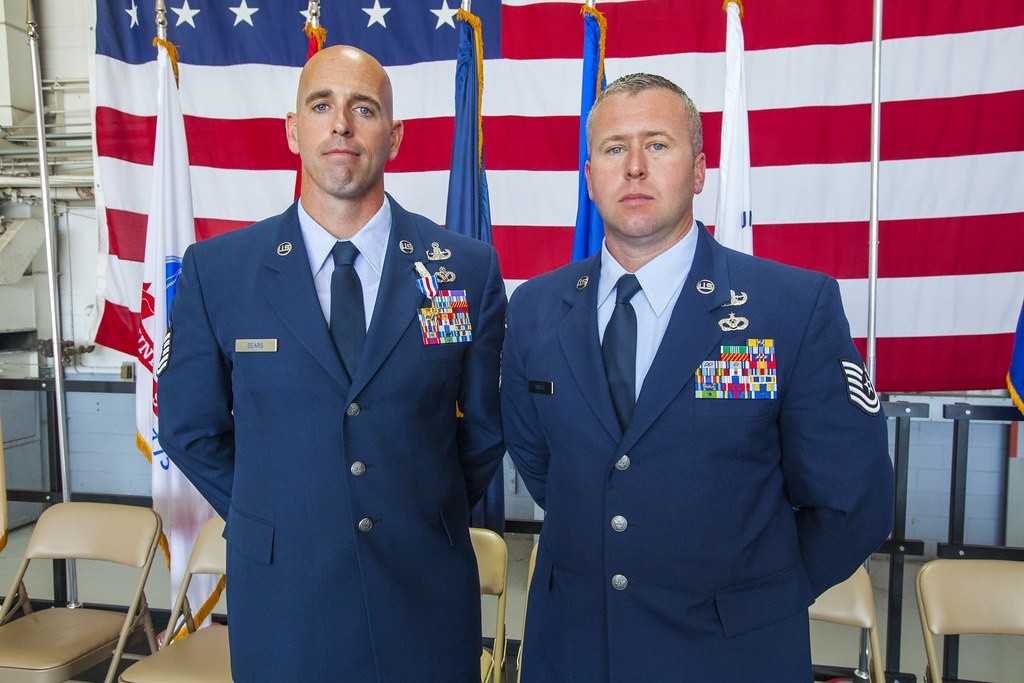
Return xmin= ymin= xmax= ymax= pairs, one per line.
xmin=159 ymin=44 xmax=508 ymax=683
xmin=498 ymin=73 xmax=900 ymax=683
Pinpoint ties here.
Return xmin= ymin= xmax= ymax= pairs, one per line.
xmin=329 ymin=240 xmax=366 ymax=385
xmin=601 ymin=274 xmax=642 ymax=436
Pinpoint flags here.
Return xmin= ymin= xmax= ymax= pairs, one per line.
xmin=446 ymin=8 xmax=492 ymax=245
xmin=88 ymin=0 xmax=1024 ymax=394
xmin=136 ymin=41 xmax=216 ymax=639
xmin=569 ymin=5 xmax=605 ymax=264
xmin=714 ymin=0 xmax=753 ymax=256
xmin=1006 ymin=299 xmax=1024 ymax=416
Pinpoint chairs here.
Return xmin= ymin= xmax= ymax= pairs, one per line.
xmin=808 ymin=564 xmax=888 ymax=683
xmin=0 ymin=502 xmax=163 ymax=683
xmin=916 ymin=559 xmax=1024 ymax=683
xmin=119 ymin=515 xmax=235 ymax=683
xmin=467 ymin=527 xmax=540 ymax=683
